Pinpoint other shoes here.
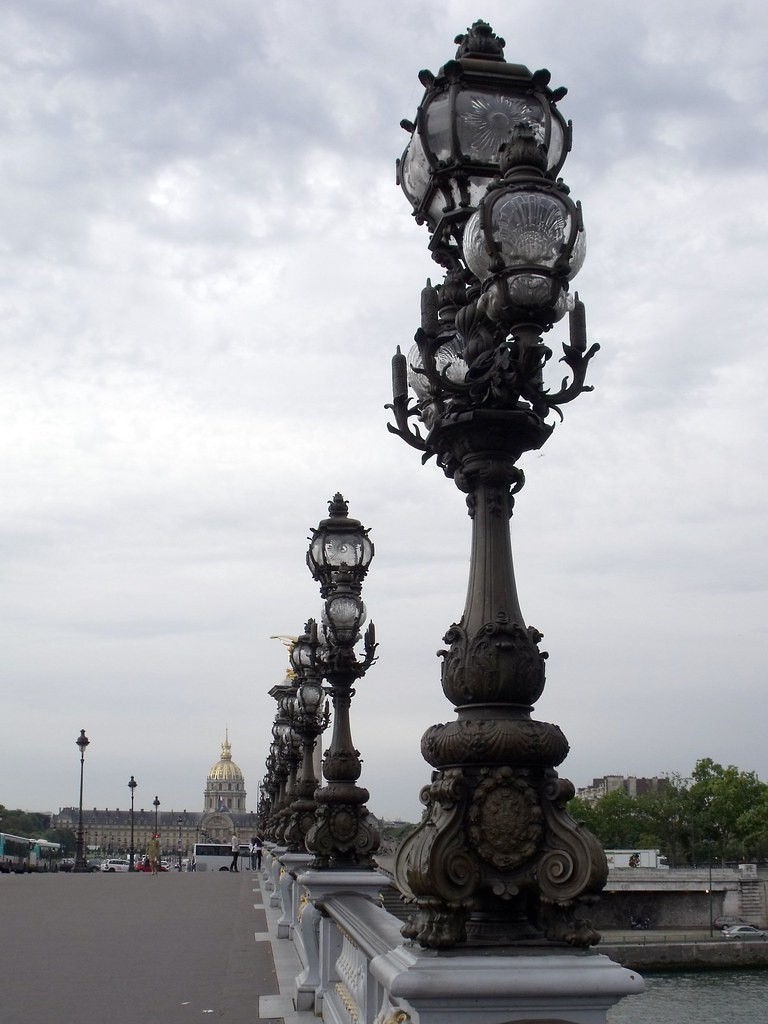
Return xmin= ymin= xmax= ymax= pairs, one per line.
xmin=252 ymin=867 xmax=256 ymax=870
xmin=230 ymin=870 xmax=234 ymax=872
xmin=235 ymin=869 xmax=240 ymax=872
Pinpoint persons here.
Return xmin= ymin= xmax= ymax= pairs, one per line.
xmin=146 ymin=834 xmax=160 ymax=875
xmin=249 ymin=831 xmax=265 ymax=870
xmin=230 ymin=832 xmax=240 ymax=872
xmin=187 ymin=856 xmax=197 ymax=873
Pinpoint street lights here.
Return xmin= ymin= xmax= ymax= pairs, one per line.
xmin=306 ymin=492 xmax=380 ymax=873
xmin=177 ymin=816 xmax=223 ymax=872
xmin=153 ymin=796 xmax=159 ymax=840
xmin=256 ymin=618 xmax=326 ymax=850
xmin=71 ymin=730 xmax=90 ymax=872
xmin=127 ymin=776 xmax=137 ymax=871
xmin=386 ymin=20 xmax=609 ymax=957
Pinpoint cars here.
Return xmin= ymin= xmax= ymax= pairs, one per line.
xmin=61 ymin=854 xmax=190 ymax=872
xmin=100 ymin=859 xmax=134 ymax=872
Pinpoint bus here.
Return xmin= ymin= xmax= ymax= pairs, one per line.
xmin=0 ymin=832 xmax=61 ymax=873
xmin=191 ymin=844 xmax=259 ymax=872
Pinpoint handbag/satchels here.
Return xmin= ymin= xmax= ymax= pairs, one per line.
xmin=249 ymin=851 xmax=253 ymax=856
